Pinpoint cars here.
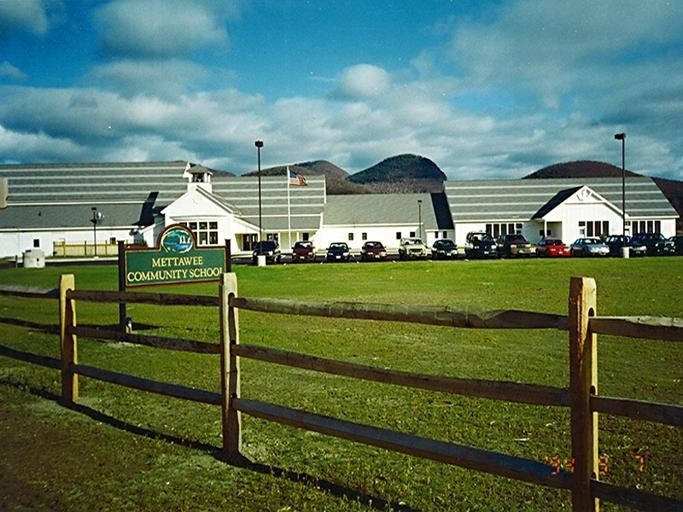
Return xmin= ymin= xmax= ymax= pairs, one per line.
xmin=288 ymin=239 xmax=316 ymax=264
xmin=429 ymin=238 xmax=460 ymax=260
xmin=358 ymin=240 xmax=387 ymax=261
xmin=324 ymin=240 xmax=352 ymax=262
xmin=533 ymin=237 xmax=570 ymax=258
xmin=568 ymin=231 xmax=682 ymax=259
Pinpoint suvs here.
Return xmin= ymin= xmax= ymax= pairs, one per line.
xmin=396 ymin=235 xmax=428 ymax=260
xmin=495 ymin=233 xmax=534 ymax=258
xmin=463 ymin=230 xmax=498 ymax=260
xmin=250 ymin=239 xmax=282 ymax=265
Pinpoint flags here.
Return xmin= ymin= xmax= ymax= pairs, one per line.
xmin=290 ymin=169 xmax=308 ymax=186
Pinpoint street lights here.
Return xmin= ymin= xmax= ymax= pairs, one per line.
xmin=612 ymin=132 xmax=630 ymax=258
xmin=415 ymin=198 xmax=422 ymax=238
xmin=89 ymin=206 xmax=99 ymax=260
xmin=253 ymin=139 xmax=266 ymax=267
